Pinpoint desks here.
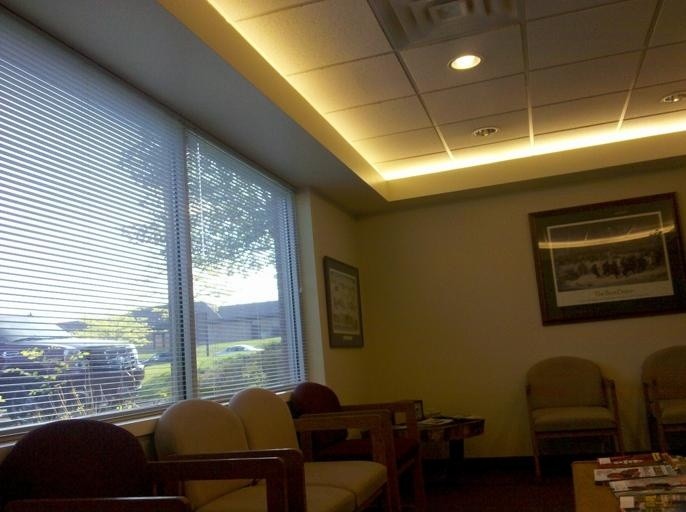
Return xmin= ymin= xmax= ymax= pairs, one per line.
xmin=569 ymin=455 xmax=685 ymax=511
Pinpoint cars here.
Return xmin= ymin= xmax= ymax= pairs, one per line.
xmin=139 ymin=345 xmax=264 ymax=365
xmin=0 ymin=313 xmax=145 ymax=418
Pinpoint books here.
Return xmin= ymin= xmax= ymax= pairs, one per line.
xmin=591 ymin=451 xmax=686 ymax=512
xmin=416 ymin=416 xmax=453 ymax=426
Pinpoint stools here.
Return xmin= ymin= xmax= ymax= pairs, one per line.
xmin=416 ymin=415 xmax=486 ymax=492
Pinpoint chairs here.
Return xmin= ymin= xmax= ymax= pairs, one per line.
xmin=0 ymin=417 xmax=289 ymax=512
xmin=287 ymin=380 xmax=424 ymax=499
xmin=230 ymin=387 xmax=396 ymax=512
xmin=155 ymin=398 xmax=357 ymax=512
xmin=523 ymin=344 xmax=685 ymax=481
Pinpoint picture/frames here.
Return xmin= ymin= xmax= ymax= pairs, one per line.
xmin=322 ymin=254 xmax=368 ymax=348
xmin=526 ymin=189 xmax=686 ymax=328
